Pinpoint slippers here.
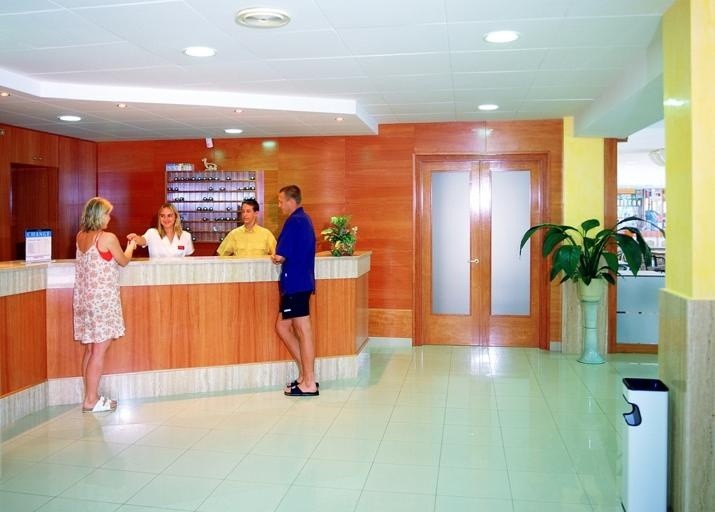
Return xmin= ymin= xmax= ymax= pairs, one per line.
xmin=288 ymin=380 xmax=318 ymax=387
xmin=82 ymin=396 xmax=117 ymax=413
xmin=285 ymin=385 xmax=319 ymax=396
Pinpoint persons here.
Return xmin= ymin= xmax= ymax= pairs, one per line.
xmin=215 ymin=198 xmax=278 ymax=257
xmin=270 ymin=184 xmax=320 ymax=396
xmin=126 ymin=203 xmax=196 ymax=257
xmin=72 ymin=196 xmax=137 ymax=413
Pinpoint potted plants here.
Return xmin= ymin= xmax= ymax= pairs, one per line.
xmin=321 ymin=215 xmax=358 ymax=257
xmin=519 ymin=216 xmax=666 ymax=365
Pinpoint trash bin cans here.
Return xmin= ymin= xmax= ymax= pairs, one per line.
xmin=619 ymin=378 xmax=669 ymax=512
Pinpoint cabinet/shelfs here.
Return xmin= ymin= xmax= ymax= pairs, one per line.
xmin=164 ymin=169 xmax=258 ymax=244
